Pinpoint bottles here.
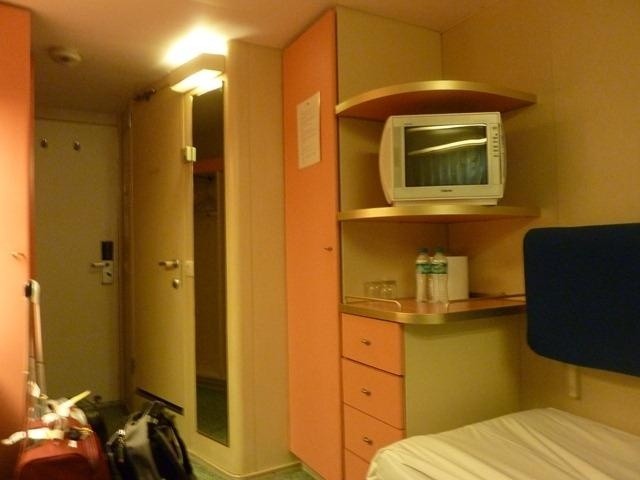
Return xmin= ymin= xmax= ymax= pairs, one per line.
xmin=414 ymin=246 xmax=449 ymax=304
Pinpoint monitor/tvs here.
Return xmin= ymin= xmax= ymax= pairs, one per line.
xmin=377 ymin=111 xmax=507 ymax=206
xmin=521 ymin=222 xmax=640 ymax=379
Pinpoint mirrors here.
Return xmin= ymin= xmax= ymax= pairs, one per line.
xmin=190 ymin=79 xmax=228 ymax=447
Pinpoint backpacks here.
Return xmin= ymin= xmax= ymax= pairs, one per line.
xmin=105 ymin=402 xmax=198 ymax=480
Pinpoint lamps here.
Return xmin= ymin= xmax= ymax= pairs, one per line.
xmin=193 ymin=80 xmax=223 ymax=98
xmin=169 ymin=53 xmax=226 ymax=95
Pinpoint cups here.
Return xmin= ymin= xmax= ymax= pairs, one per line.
xmin=363 ymin=280 xmax=399 ymax=301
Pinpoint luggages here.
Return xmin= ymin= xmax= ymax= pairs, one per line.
xmin=16 ymin=394 xmax=106 ymax=479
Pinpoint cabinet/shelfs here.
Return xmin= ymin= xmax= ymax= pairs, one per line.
xmin=282 ymin=7 xmax=541 ymax=480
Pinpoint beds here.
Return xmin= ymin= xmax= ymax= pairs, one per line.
xmin=364 ymin=406 xmax=640 ymax=480
